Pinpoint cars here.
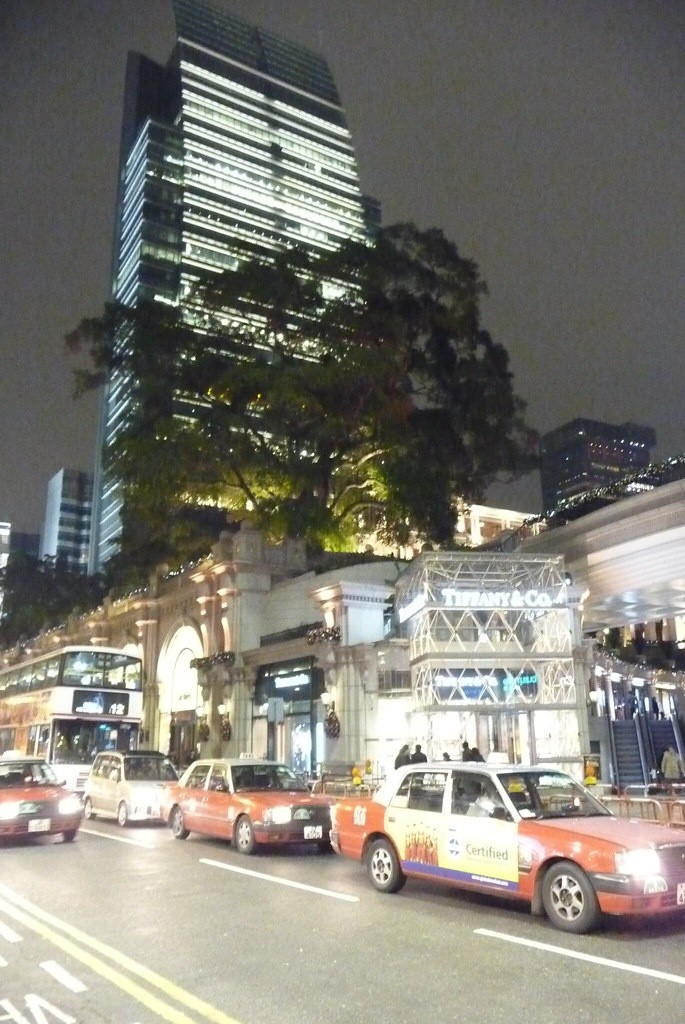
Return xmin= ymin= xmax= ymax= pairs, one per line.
xmin=82 ymin=750 xmax=181 ymax=828
xmin=162 ymin=752 xmax=336 ymax=856
xmin=327 ymin=751 xmax=685 ymax=934
xmin=0 ymin=749 xmax=84 ymax=843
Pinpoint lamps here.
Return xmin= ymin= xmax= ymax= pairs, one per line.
xmin=320 ymin=692 xmax=334 ymax=718
xmin=218 ymin=700 xmax=229 ymax=727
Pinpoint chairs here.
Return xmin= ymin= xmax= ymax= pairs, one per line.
xmin=508 ymin=792 xmax=536 ymax=811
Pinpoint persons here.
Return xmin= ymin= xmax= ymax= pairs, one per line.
xmin=658 ymin=744 xmax=685 ymax=802
xmin=443 ymin=753 xmax=451 ymax=761
xmin=626 ymin=690 xmax=638 ymax=719
xmin=411 ymin=745 xmax=427 ymax=763
xmin=471 ymin=748 xmax=485 ymax=762
xmin=462 ymin=742 xmax=473 ymax=761
xmin=652 ymin=696 xmax=659 ymax=720
xmin=395 ymin=745 xmax=412 ymax=770
xmin=186 ymin=749 xmax=197 ymax=766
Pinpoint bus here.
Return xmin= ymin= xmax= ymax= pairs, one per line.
xmin=0 ymin=646 xmax=145 ymax=798
